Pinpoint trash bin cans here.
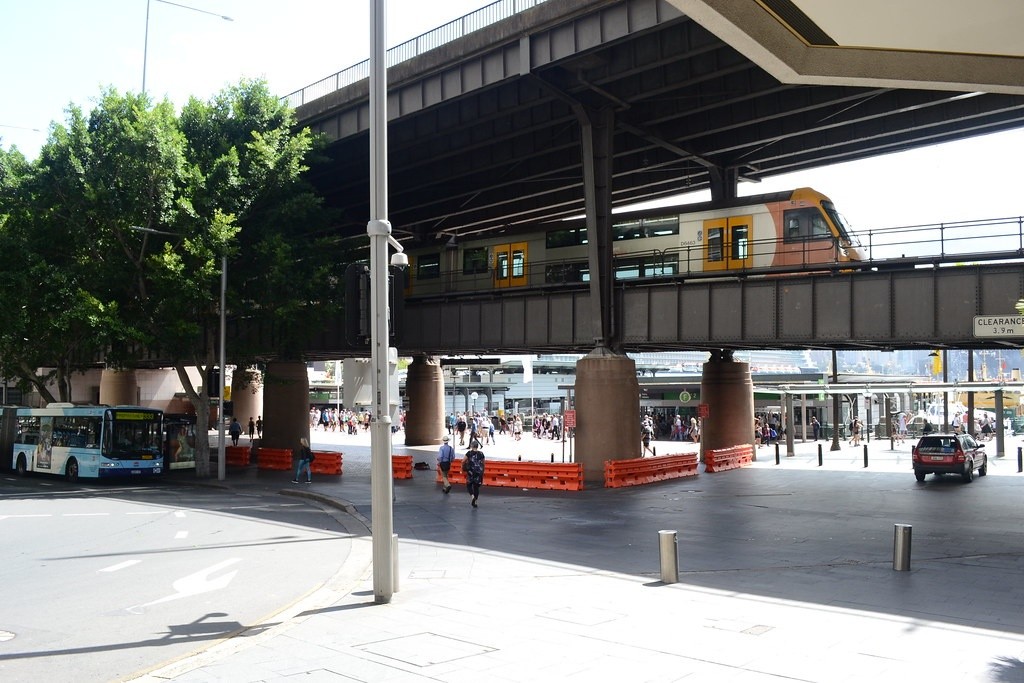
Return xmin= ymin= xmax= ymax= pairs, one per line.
xmin=251 ymin=439 xmax=263 ymax=455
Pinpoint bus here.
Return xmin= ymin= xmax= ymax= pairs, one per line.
xmin=11 ymin=403 xmax=167 ymax=484
xmin=160 ymin=414 xmax=203 ymax=470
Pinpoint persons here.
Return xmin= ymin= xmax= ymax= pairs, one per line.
xmin=953 ymin=411 xmax=968 ymax=434
xmin=657 ymin=413 xmax=683 ymax=441
xmin=460 ymin=440 xmax=485 ymax=508
xmin=499 ymin=413 xmax=523 ymax=441
xmin=891 ymin=412 xmax=907 ymax=443
xmin=755 ymin=413 xmax=787 ymax=449
xmin=292 ymin=437 xmax=312 ymax=483
xmin=681 ymin=416 xmax=701 ymax=442
xmin=175 ymin=428 xmax=192 ymax=462
xmin=449 ymin=409 xmax=496 ymax=449
xmin=923 ymin=418 xmax=934 ymax=435
xmin=310 ymin=406 xmax=321 ymax=430
xmin=849 ymin=415 xmax=864 ymax=446
xmin=810 ymin=416 xmax=820 ymax=441
xmin=533 ymin=412 xmax=560 ymax=440
xmin=357 ymin=409 xmax=371 ymax=432
xmin=247 ymin=417 xmax=255 ymax=442
xmin=322 ymin=408 xmax=358 ymax=435
xmin=641 ymin=414 xmax=654 ymax=458
xmin=229 ymin=418 xmax=243 ymax=446
xmin=974 ymin=412 xmax=994 ymax=441
xmin=436 ymin=436 xmax=455 ymax=494
xmin=256 ymin=416 xmax=263 ymax=439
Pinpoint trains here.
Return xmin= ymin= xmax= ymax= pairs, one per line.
xmin=330 ymin=186 xmax=875 ymax=296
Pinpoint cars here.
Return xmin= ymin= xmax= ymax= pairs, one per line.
xmin=906 ymin=414 xmax=956 ymax=435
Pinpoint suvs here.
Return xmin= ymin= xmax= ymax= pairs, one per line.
xmin=913 ymin=432 xmax=989 ymax=482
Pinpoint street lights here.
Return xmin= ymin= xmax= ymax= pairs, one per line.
xmin=131 ymin=225 xmax=228 ymax=481
xmin=141 ymin=0 xmax=232 ymax=93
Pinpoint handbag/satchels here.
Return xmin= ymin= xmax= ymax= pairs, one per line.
xmin=440 ymin=461 xmax=450 ymax=471
xmin=309 ymin=452 xmax=315 ymax=462
xmin=462 ymin=453 xmax=470 ymax=471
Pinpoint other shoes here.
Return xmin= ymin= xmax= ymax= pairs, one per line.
xmin=473 ymin=504 xmax=477 ymax=507
xmin=480 ymin=446 xmax=483 ymax=449
xmin=471 ymin=497 xmax=476 ymax=505
xmin=292 ymin=480 xmax=299 ymax=484
xmin=305 ymin=479 xmax=311 ymax=483
xmin=443 ymin=489 xmax=446 ymax=491
xmin=466 ymin=447 xmax=470 ymax=449
xmin=445 ymin=487 xmax=451 ymax=493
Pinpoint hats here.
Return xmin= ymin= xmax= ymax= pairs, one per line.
xmin=442 ymin=436 xmax=451 ymax=443
xmin=642 ymin=421 xmax=650 ymax=425
